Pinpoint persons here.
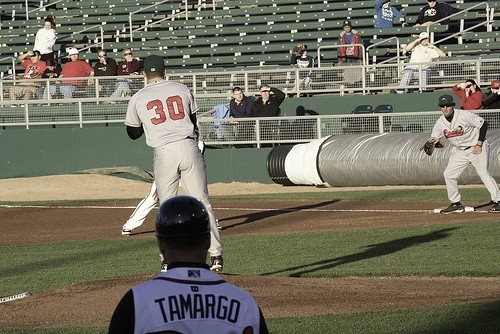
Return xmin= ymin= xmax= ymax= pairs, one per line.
xmin=336 ymin=19 xmax=365 ymax=95
xmin=228 ymin=84 xmax=286 ymax=150
xmin=395 ymin=31 xmax=446 ymax=94
xmin=287 ymin=44 xmax=315 ymax=98
xmin=427 ymin=93 xmax=500 ymax=215
xmin=9 ymin=46 xmax=142 ymax=108
xmin=452 ymin=79 xmax=484 ymax=116
xmin=123 ymin=54 xmax=226 ymax=274
xmin=372 ymin=0 xmax=406 ymax=58
xmin=108 ymin=195 xmax=270 ymax=334
xmin=482 ymin=80 xmax=500 ymax=125
xmin=32 ymin=16 xmax=58 ymax=61
xmin=119 ymin=139 xmax=221 ymax=236
xmin=411 ymin=0 xmax=469 ymax=45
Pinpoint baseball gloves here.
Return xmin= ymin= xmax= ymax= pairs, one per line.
xmin=424 ymin=138 xmax=445 ymax=156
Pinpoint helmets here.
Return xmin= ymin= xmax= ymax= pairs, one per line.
xmin=154 ymin=196 xmax=211 ymax=238
xmin=438 ymin=94 xmax=456 ymax=106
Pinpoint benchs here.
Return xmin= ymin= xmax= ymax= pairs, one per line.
xmin=0 ymin=0 xmax=500 ymax=110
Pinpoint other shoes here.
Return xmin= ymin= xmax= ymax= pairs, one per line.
xmin=122 ymin=229 xmax=130 ymax=236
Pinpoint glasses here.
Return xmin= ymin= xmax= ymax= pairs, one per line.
xmin=440 ymin=105 xmax=451 ymax=108
xmin=428 ymin=0 xmax=436 ymax=2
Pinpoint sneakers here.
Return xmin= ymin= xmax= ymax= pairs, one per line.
xmin=209 ymin=256 xmax=223 ymax=271
xmin=441 ymin=203 xmax=464 ymax=214
xmin=160 ymin=263 xmax=167 ymax=272
xmin=488 ymin=204 xmax=500 ymax=212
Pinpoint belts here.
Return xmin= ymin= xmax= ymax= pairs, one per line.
xmin=186 ymin=136 xmax=195 ymax=139
xmin=464 ymin=146 xmax=471 ymax=150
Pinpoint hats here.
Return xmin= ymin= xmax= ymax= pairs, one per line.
xmin=69 ymin=49 xmax=79 ymax=55
xmin=143 ymin=55 xmax=164 ymax=72
xmin=343 ymin=21 xmax=351 ymax=26
xmin=491 ymin=80 xmax=500 ymax=88
xmin=419 ymin=32 xmax=430 ymax=39
